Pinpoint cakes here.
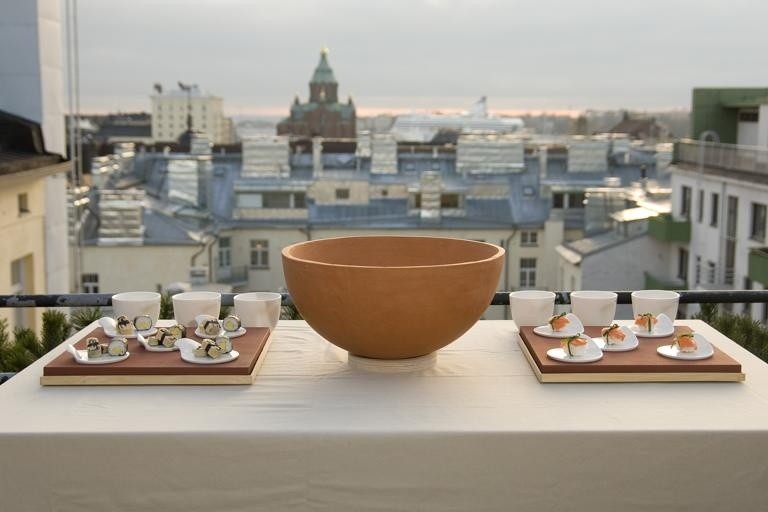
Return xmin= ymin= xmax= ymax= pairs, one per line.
xmin=547 ymin=309 xmax=704 ymax=360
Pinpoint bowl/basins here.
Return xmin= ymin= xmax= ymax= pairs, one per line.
xmin=279 ymin=234 xmax=509 ymax=361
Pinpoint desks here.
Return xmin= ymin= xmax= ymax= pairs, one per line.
xmin=0 ymin=311 xmax=767 ymax=511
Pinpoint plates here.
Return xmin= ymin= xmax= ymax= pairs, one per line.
xmin=195 ymin=315 xmax=246 ymax=340
xmin=656 ymin=332 xmax=713 ymax=361
xmin=548 ymin=334 xmax=604 ymax=363
xmin=98 ymin=318 xmax=158 ymax=339
xmin=533 ymin=312 xmax=584 ymax=338
xmin=137 ymin=334 xmax=183 ymax=351
xmin=628 ymin=314 xmax=674 ymax=338
xmin=65 ymin=344 xmax=131 ymax=366
xmin=590 ymin=324 xmax=641 ymax=352
xmin=173 ymin=337 xmax=239 ymax=365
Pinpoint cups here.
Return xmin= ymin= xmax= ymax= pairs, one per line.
xmin=111 ymin=290 xmax=162 ymax=325
xmin=172 ymin=291 xmax=222 ymax=325
xmin=571 ymin=291 xmax=618 ymax=329
xmin=231 ymin=292 xmax=283 ymax=334
xmin=507 ymin=290 xmax=555 ymax=325
xmin=629 ymin=288 xmax=681 ymax=324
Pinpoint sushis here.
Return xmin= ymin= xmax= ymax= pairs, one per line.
xmin=82 ymin=311 xmax=246 ymax=361
